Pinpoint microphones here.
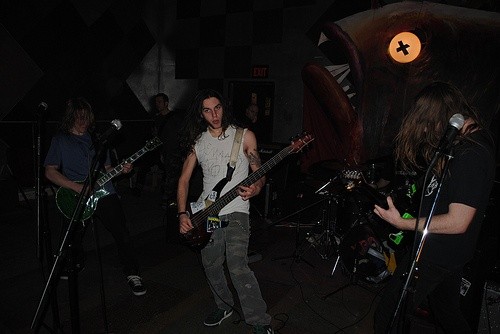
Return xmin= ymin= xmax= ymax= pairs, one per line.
xmin=430 ymin=113 xmax=464 ymax=167
xmin=89 ymin=120 xmax=122 ymax=152
xmin=38 ymin=102 xmax=48 ymax=121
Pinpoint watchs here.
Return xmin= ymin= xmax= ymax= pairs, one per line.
xmin=177 ymin=212 xmax=186 ymax=218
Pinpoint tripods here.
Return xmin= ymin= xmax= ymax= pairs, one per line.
xmin=272 ymin=196 xmax=345 ymax=268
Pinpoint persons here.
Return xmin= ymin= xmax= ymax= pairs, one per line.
xmin=372 ymin=83 xmax=494 ymax=334
xmin=368 ymin=141 xmax=422 ymax=257
xmin=143 ymin=93 xmax=259 ymax=209
xmin=43 ymin=97 xmax=146 ymax=296
xmin=177 ymin=90 xmax=275 ymax=334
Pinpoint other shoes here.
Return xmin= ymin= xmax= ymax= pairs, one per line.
xmin=125 ymin=272 xmax=147 ymax=295
xmin=59 ymin=264 xmax=84 ymax=279
xmin=254 ymin=326 xmax=274 ymax=334
xmin=203 ymin=309 xmax=233 ymax=326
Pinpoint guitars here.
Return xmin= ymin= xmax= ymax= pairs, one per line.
xmin=342 ymin=169 xmax=418 ymax=250
xmin=182 ymin=130 xmax=316 ymax=251
xmin=56 ymin=136 xmax=164 ymax=222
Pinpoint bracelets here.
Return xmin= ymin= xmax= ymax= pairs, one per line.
xmin=375 ymin=184 xmax=379 ymax=190
